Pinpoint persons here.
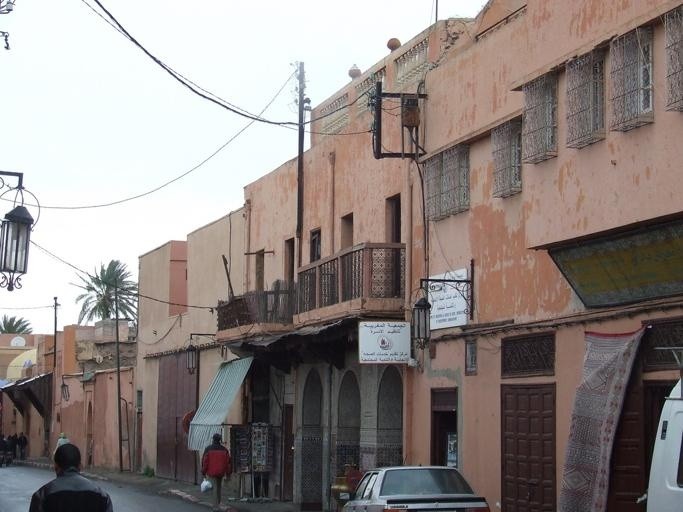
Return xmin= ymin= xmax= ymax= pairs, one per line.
xmin=51 ymin=432 xmax=69 ymax=471
xmin=0 ymin=431 xmax=26 ymax=467
xmin=201 ymin=432 xmax=231 ymax=510
xmin=28 ymin=442 xmax=112 ymax=510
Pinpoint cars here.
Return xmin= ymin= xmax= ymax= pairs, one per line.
xmin=335 ymin=463 xmax=490 ymax=512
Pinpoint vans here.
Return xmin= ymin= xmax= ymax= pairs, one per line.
xmin=647 ymin=376 xmax=683 ymax=512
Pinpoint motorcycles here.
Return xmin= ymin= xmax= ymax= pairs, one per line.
xmin=0 ymin=449 xmax=15 ymax=469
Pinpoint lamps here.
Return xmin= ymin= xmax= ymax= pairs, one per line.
xmin=184 ymin=331 xmax=228 ymax=376
xmin=412 ymin=275 xmax=472 ymax=351
xmin=0 ymin=167 xmax=36 ymax=293
xmin=60 ymin=370 xmax=84 ymax=404
